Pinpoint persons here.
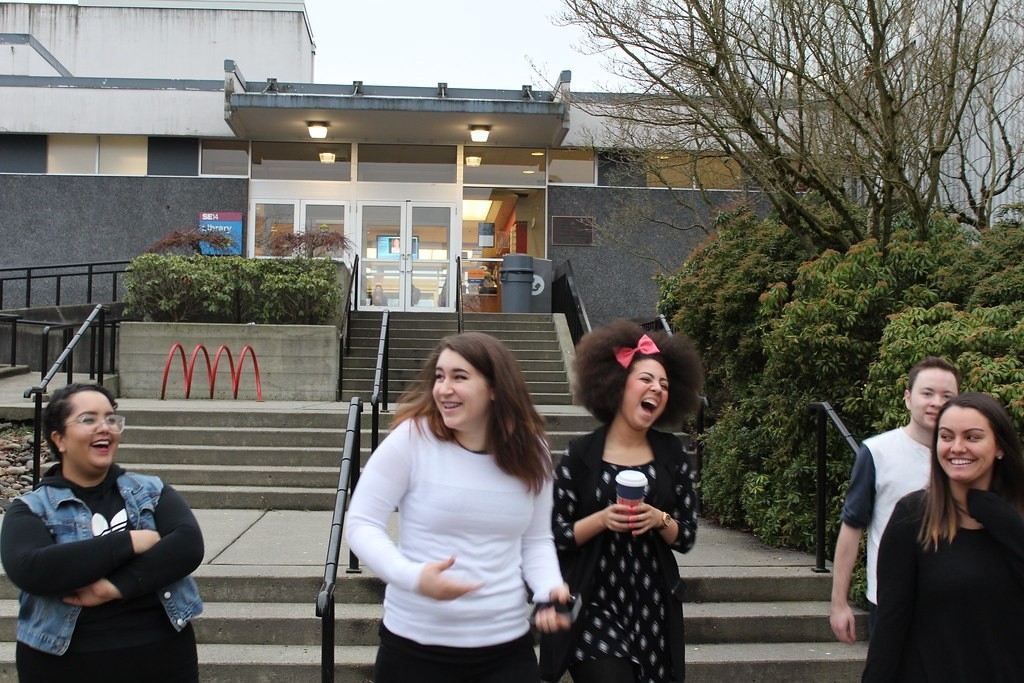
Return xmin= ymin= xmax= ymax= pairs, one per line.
xmin=411 ymin=284 xmax=420 ymax=306
xmin=344 ymin=319 xmax=706 ymax=683
xmin=391 ymin=238 xmax=400 ymax=253
xmin=829 ymin=356 xmax=1024 ymax=683
xmin=0 ymin=383 xmax=206 ymax=683
xmin=372 ymin=285 xmax=388 ymax=306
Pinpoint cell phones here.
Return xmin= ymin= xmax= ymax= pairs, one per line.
xmin=528 ymin=593 xmax=582 ymax=625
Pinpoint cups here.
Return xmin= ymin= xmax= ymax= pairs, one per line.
xmin=615 ymin=470 xmax=648 ymax=515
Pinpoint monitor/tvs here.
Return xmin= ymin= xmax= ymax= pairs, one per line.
xmin=376 ymin=234 xmax=419 ymax=261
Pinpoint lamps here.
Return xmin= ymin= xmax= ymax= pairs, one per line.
xmin=319 ymin=149 xmax=336 ymax=164
xmin=308 ymin=121 xmax=328 ymax=138
xmin=466 ymin=153 xmax=482 ymax=168
xmin=470 ymin=125 xmax=490 ymax=143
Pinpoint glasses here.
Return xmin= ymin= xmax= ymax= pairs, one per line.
xmin=62 ymin=414 xmax=126 ymax=434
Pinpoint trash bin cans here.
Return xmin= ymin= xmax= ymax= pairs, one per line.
xmin=497 ymin=253 xmax=535 ymax=313
xmin=531 ymin=257 xmax=553 ymax=313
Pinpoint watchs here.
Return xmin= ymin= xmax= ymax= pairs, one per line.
xmin=656 ymin=511 xmax=671 ymax=531
